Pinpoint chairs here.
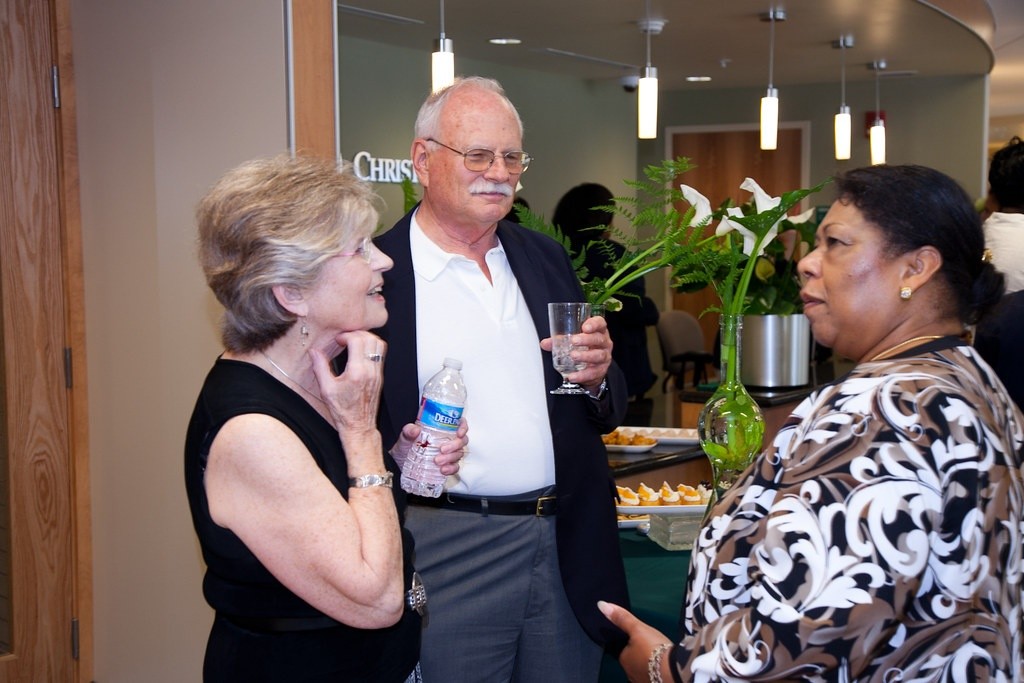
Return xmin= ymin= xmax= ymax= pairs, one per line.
xmin=658 ymin=309 xmax=721 ymax=395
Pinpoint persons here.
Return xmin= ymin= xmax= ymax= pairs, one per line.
xmin=597 ymin=164 xmax=1024 ymax=683
xmin=328 ymin=77 xmax=630 ymax=683
xmin=979 ymin=136 xmax=1024 ymax=416
xmin=503 ymin=182 xmax=659 ymax=426
xmin=184 ymin=152 xmax=421 ymax=683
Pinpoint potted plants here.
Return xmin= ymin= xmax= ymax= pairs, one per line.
xmin=711 ymin=194 xmax=822 ymax=392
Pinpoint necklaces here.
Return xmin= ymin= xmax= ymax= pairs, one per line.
xmin=871 ymin=335 xmax=943 ymax=362
xmin=259 ymin=349 xmax=328 ymax=406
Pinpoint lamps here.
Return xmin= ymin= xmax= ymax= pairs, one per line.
xmin=867 ymin=59 xmax=886 ymax=166
xmin=760 ymin=9 xmax=785 ymax=150
xmin=431 ymin=0 xmax=455 ymax=94
xmin=637 ymin=0 xmax=658 ymax=140
xmin=830 ymin=36 xmax=855 ymax=161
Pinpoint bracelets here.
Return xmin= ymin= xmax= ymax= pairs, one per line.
xmin=647 ymin=643 xmax=674 ymax=683
xmin=585 ymin=377 xmax=607 ymax=399
xmin=345 ymin=473 xmax=394 ymax=489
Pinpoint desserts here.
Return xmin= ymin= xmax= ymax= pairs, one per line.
xmin=615 ymin=479 xmax=713 ymax=507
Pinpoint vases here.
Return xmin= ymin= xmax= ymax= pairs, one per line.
xmin=697 ymin=313 xmax=767 ymax=526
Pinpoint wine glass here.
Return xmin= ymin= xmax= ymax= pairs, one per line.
xmin=547 ymin=302 xmax=591 ymax=395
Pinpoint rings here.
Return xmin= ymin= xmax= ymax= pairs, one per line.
xmin=369 ymin=354 xmax=380 ymax=361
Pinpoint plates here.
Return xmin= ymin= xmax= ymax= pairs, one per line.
xmin=614 ymin=496 xmax=710 ymax=514
xmin=599 ymin=435 xmax=660 ymax=453
xmin=614 ymin=427 xmax=702 ymax=442
xmin=618 ymin=519 xmax=651 ymax=528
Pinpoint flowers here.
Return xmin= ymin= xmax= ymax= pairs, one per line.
xmin=511 ymin=145 xmax=842 ymax=314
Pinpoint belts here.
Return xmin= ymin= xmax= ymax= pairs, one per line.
xmin=406 ymin=488 xmax=560 ymax=516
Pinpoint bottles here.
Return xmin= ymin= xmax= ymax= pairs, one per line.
xmin=399 ymin=358 xmax=468 ymax=498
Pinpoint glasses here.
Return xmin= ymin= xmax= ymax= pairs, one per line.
xmin=426 ymin=135 xmax=533 ymax=176
